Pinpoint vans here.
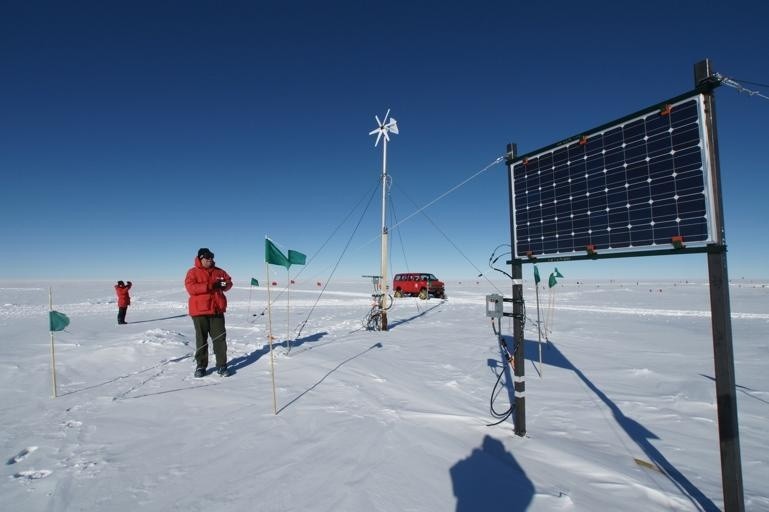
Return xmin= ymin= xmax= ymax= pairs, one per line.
xmin=394 ymin=273 xmax=444 ymax=300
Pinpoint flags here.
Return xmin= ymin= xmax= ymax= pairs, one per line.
xmin=265 ymin=238 xmax=292 ymax=271
xmin=251 ymin=278 xmax=259 ymax=286
xmin=533 ymin=265 xmax=540 ymax=284
xmin=555 ymin=268 xmax=564 ymax=278
xmin=48 ymin=311 xmax=70 ymax=331
xmin=548 ymin=272 xmax=557 ymax=288
xmin=287 ymin=249 xmax=307 ymax=265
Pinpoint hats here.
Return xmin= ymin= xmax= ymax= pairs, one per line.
xmin=197 ymin=247 xmax=215 ymax=259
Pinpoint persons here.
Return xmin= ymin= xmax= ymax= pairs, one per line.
xmin=184 ymin=248 xmax=233 ymax=378
xmin=113 ymin=280 xmax=133 ymax=324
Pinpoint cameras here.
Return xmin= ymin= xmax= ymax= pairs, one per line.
xmin=218 ymin=279 xmax=227 ymax=288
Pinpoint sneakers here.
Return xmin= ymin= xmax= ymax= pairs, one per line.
xmin=194 ymin=366 xmax=231 ymax=379
xmin=118 ymin=321 xmax=127 ymax=324
